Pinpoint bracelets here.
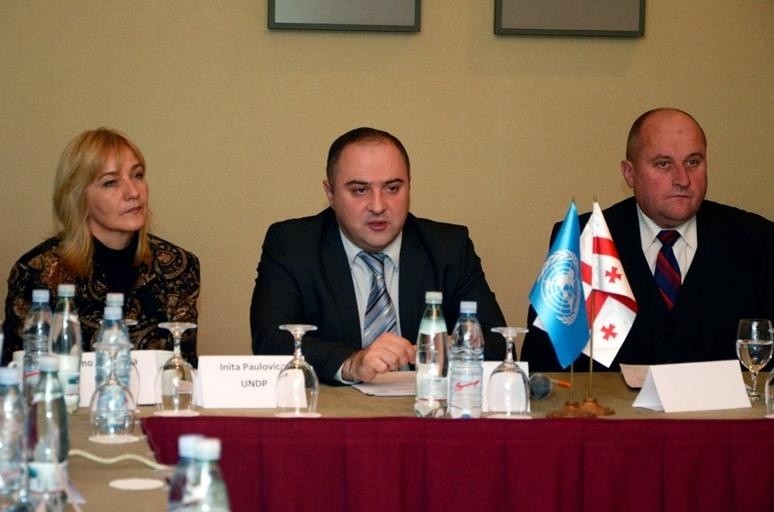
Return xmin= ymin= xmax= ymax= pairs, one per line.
xmin=349 ymin=349 xmax=363 ymax=384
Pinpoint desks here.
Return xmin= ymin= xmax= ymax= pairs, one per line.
xmin=0 ymin=367 xmax=773 ymax=511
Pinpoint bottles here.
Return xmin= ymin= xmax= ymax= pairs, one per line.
xmin=166 ymin=434 xmax=205 ymax=512
xmin=185 ymin=438 xmax=232 ymax=511
xmin=95 ymin=307 xmax=132 ymax=434
xmin=21 ymin=290 xmax=54 ymax=406
xmin=413 ymin=290 xmax=448 ymax=418
xmin=106 ymin=293 xmax=130 ymax=342
xmin=27 ymin=355 xmax=69 ymax=512
xmin=0 ymin=366 xmax=27 ymax=512
xmin=445 ymin=300 xmax=485 ymax=420
xmin=47 ymin=283 xmax=81 ymax=415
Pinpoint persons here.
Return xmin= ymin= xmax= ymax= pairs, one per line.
xmin=0 ymin=126 xmax=200 ymax=369
xmin=520 ymin=108 xmax=774 ymax=374
xmin=250 ymin=127 xmax=516 ymax=387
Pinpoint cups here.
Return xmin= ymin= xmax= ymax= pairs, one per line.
xmin=9 ymin=351 xmax=24 ymax=393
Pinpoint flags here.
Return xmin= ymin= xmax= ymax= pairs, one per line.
xmin=532 ymin=201 xmax=641 ymax=369
xmin=526 ymin=199 xmax=590 ymax=371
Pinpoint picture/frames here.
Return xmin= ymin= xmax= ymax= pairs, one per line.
xmin=495 ymin=0 xmax=647 ymax=37
xmin=268 ymin=0 xmax=421 ymax=33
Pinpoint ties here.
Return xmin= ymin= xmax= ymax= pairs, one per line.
xmin=655 ymin=230 xmax=682 ymax=306
xmin=358 ymin=251 xmax=400 ymax=348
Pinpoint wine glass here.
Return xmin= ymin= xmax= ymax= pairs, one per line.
xmin=276 ymin=323 xmax=321 ymax=417
xmin=486 ymin=326 xmax=531 ymax=420
xmin=764 ymin=369 xmax=774 ymax=416
xmin=155 ymin=321 xmax=203 ymax=413
xmin=736 ymin=318 xmax=774 ymax=403
xmin=89 ymin=342 xmax=137 ymax=435
